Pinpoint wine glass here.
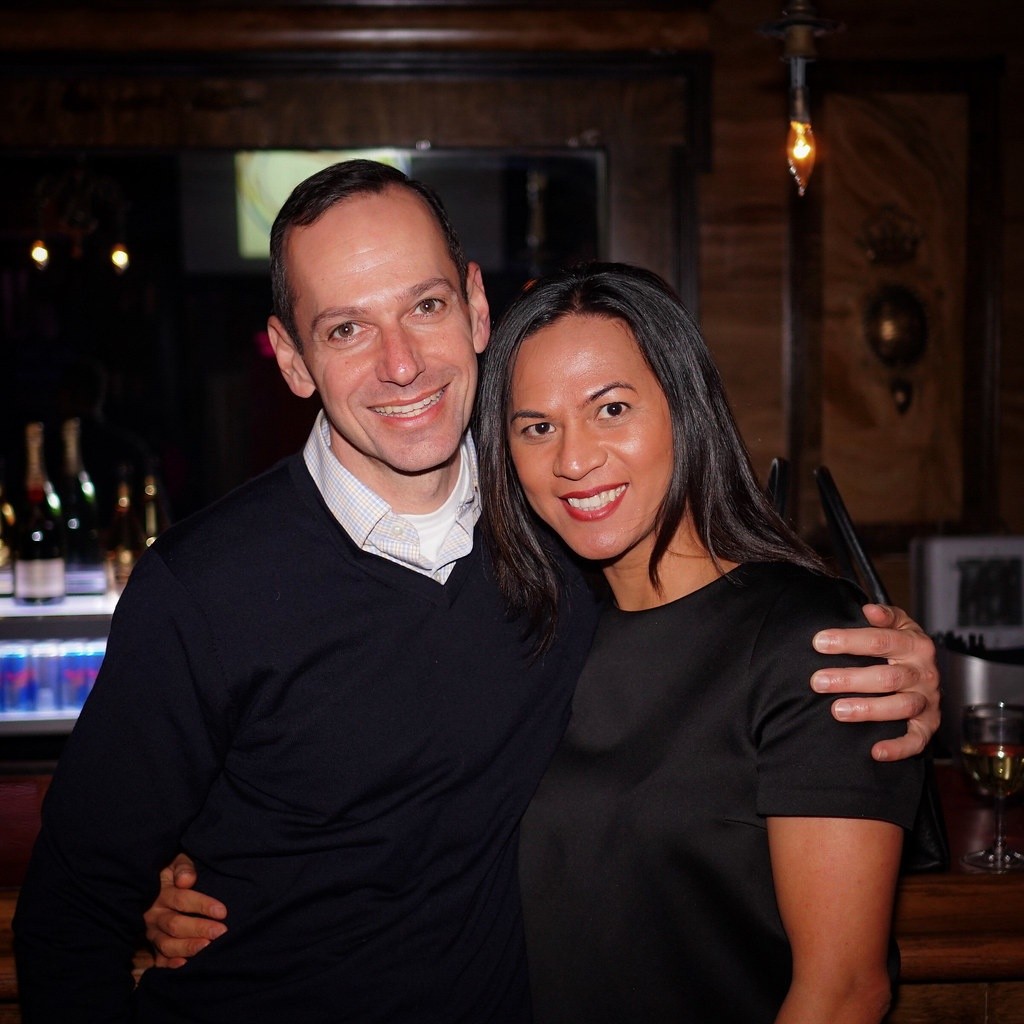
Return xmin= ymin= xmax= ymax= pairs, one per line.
xmin=959 ymin=703 xmax=1023 ymax=872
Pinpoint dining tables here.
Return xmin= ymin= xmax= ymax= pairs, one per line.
xmin=882 ymin=750 xmax=1024 ymax=1024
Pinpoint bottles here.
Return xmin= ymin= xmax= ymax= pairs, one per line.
xmin=1 ymin=413 xmax=175 ymax=606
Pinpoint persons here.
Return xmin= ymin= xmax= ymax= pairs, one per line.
xmin=142 ymin=261 xmax=930 ymax=1022
xmin=4 ymin=160 xmax=945 ymax=1023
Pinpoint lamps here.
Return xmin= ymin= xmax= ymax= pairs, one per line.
xmin=778 ymin=2 xmax=827 ymax=199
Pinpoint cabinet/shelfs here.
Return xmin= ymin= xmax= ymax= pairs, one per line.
xmin=0 ymin=588 xmax=119 ymax=735
